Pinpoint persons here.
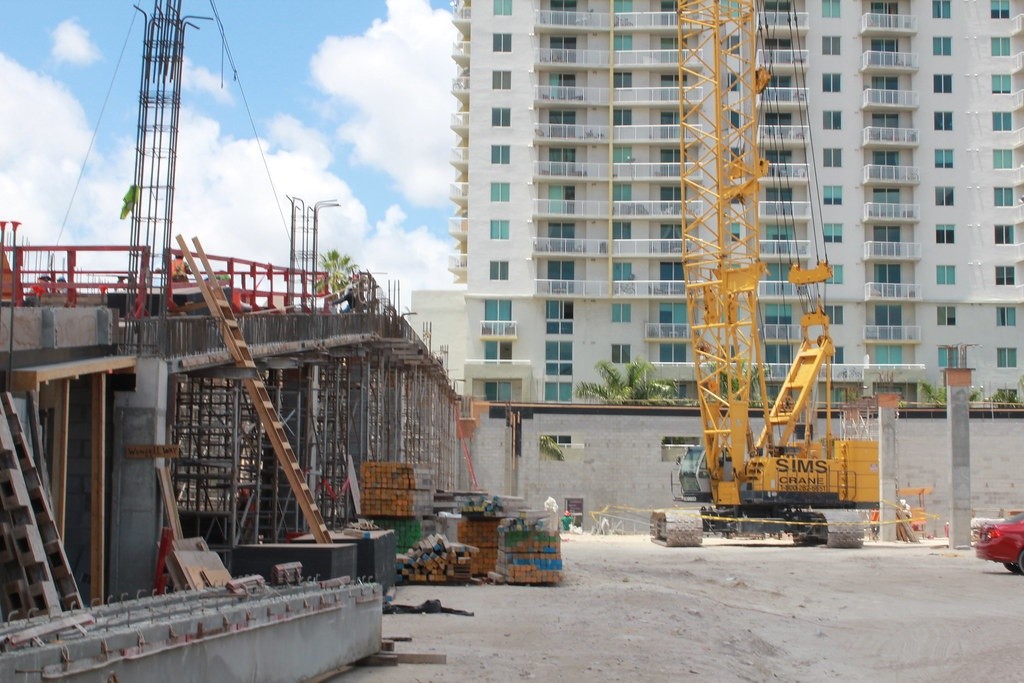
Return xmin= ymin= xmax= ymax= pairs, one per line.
xmin=332 ymin=288 xmax=357 ymax=314
xmin=172 ymin=256 xmax=192 ymax=306
xmin=561 ymin=510 xmax=573 ymax=530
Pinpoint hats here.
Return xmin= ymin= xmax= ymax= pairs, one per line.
xmin=564 ymin=511 xmax=571 ymax=516
xmin=57 ymin=276 xmax=66 ymax=283
xmin=39 ymin=275 xmax=51 ymax=281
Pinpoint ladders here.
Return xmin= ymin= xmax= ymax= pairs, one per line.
xmin=176 ymin=234 xmax=333 ymax=544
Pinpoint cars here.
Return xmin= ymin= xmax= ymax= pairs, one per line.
xmin=976 ymin=512 xmax=1024 ymax=576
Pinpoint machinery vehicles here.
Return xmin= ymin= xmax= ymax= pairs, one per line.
xmin=652 ymin=0 xmax=880 ymax=549
